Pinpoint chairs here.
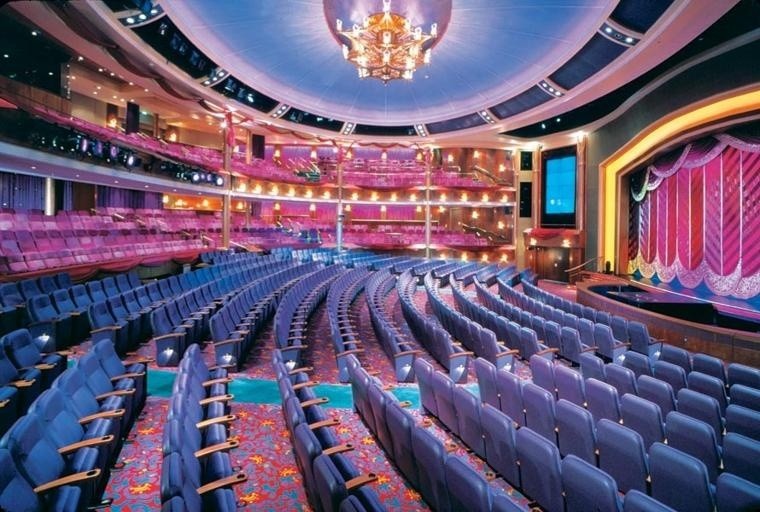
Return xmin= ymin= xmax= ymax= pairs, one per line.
xmin=0 ymin=207 xmax=760 ymax=512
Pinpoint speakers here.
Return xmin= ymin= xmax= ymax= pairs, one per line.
xmin=125 ymin=101 xmax=139 ymax=134
xmin=250 ymin=133 xmax=265 ymax=161
xmin=521 ymin=151 xmax=531 ymax=170
xmin=519 ymin=182 xmax=532 ymax=217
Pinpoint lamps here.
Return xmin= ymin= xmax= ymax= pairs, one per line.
xmin=181 ymin=169 xmax=224 ymax=187
xmin=333 ymin=0 xmax=439 ymax=81
xmin=38 ymin=127 xmax=134 ymax=168
